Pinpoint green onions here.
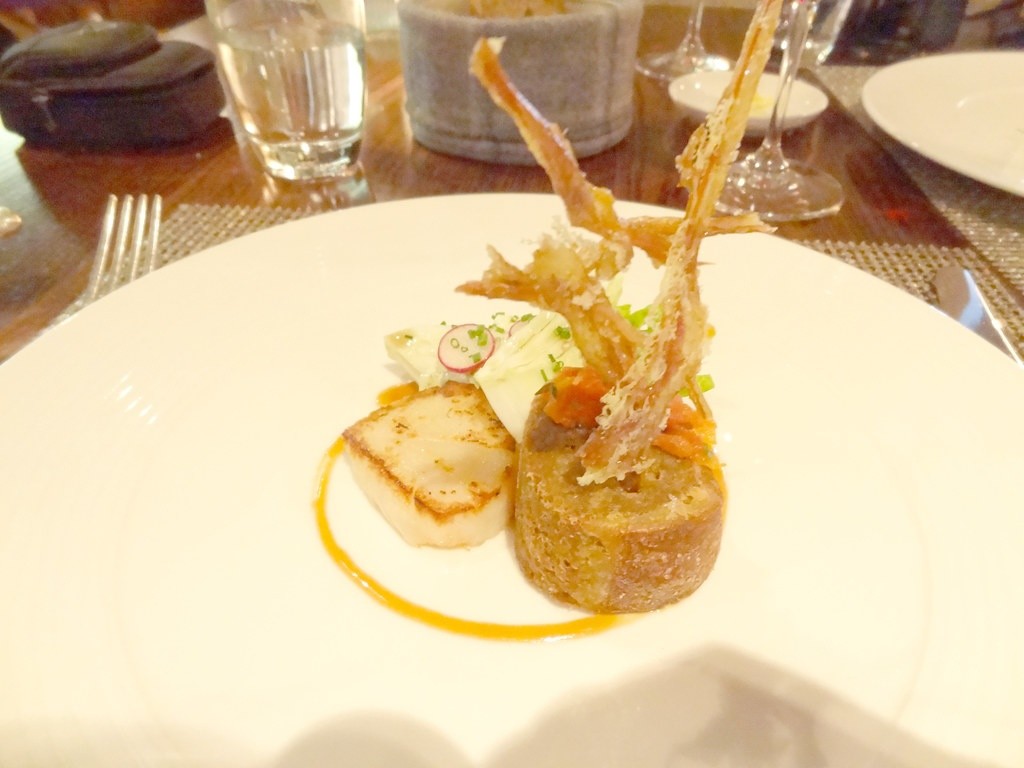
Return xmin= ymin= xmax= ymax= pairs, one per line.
xmin=437 ymin=311 xmax=570 ymax=384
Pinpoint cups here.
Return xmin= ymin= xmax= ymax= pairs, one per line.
xmin=203 ymin=0 xmax=365 ymax=179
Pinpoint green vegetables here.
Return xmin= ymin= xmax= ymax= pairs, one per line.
xmin=618 ymin=303 xmax=716 ymax=397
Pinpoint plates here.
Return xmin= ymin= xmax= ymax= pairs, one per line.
xmin=667 ymin=69 xmax=830 ymax=138
xmin=860 ymin=48 xmax=1024 ymax=205
xmin=0 ymin=191 xmax=1024 ymax=768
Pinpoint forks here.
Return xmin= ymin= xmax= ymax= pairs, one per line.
xmin=37 ymin=191 xmax=165 ymax=347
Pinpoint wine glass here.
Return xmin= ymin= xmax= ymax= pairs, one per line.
xmin=634 ymin=0 xmax=731 ymax=84
xmin=708 ymin=1 xmax=861 ymax=223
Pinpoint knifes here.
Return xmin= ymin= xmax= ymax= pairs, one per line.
xmin=937 ymin=265 xmax=1020 ymax=370
